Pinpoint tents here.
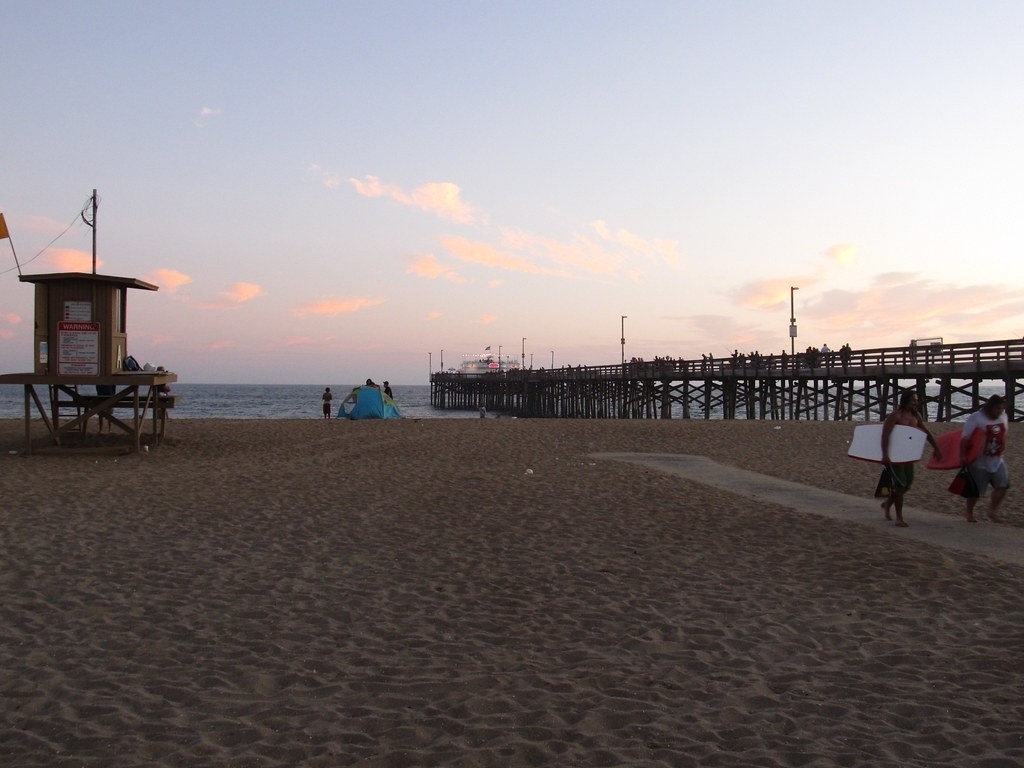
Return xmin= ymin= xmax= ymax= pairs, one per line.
xmin=335 ymin=386 xmax=403 ymax=419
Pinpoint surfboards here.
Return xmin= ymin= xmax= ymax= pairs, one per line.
xmin=924 ymin=427 xmax=988 ymax=470
xmin=847 ymin=423 xmax=927 ymax=464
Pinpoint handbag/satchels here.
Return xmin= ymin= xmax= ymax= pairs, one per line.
xmin=874 ymin=468 xmax=894 ymax=498
xmin=947 ymin=465 xmax=979 ymax=499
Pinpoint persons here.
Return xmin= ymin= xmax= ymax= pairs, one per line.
xmin=485 ymin=343 xmax=851 ymax=379
xmin=479 ymin=403 xmax=486 ymax=418
xmin=322 ymin=388 xmax=332 ymax=419
xmin=157 ymin=366 xmax=169 ymax=420
xmin=880 ymin=390 xmax=943 ymax=527
xmin=96 ymin=385 xmax=116 ymax=433
xmin=959 ymin=394 xmax=1009 ymax=522
xmin=383 ymin=381 xmax=393 ymax=400
xmin=366 ymin=379 xmax=375 ymax=385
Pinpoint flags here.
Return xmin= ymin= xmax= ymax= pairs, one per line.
xmin=0 ymin=214 xmax=9 ymax=240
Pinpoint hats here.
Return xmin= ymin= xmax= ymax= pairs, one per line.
xmin=383 ymin=381 xmax=389 ymax=385
